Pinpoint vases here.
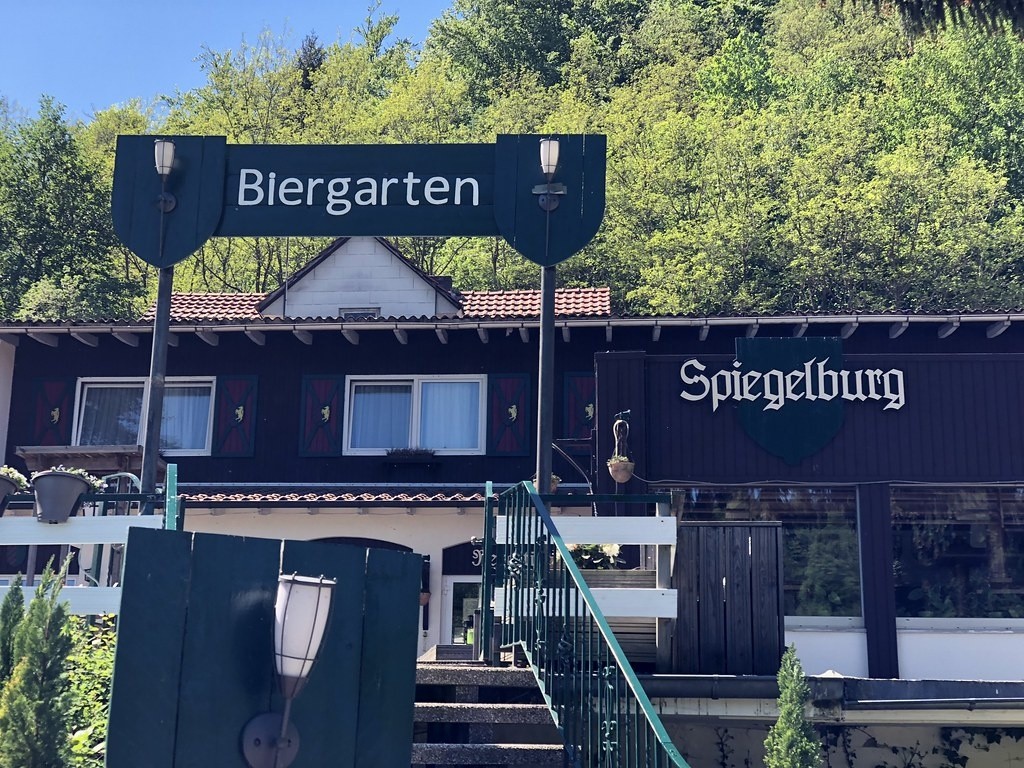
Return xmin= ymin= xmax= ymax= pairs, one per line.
xmin=0 ymin=475 xmax=19 ymax=507
xmin=502 ymin=568 xmax=655 ymax=663
xmin=30 ymin=472 xmax=91 ymax=523
xmin=608 ymin=462 xmax=635 ymax=483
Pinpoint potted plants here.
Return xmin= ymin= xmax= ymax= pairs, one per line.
xmin=532 ymin=472 xmax=561 ymax=493
xmin=384 ymin=445 xmax=436 ymax=464
xmin=420 ymin=588 xmax=431 ymax=606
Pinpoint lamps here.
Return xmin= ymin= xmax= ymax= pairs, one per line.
xmin=535 ymin=135 xmax=562 ymax=257
xmin=152 ymin=137 xmax=176 ymax=258
xmin=239 ymin=571 xmax=337 ymax=768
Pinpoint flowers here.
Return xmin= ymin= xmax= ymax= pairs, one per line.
xmin=606 ymin=455 xmax=628 ymax=466
xmin=0 ymin=464 xmax=30 ymax=490
xmin=29 ymin=463 xmax=108 ymax=512
xmin=549 ymin=543 xmax=627 ymax=570
